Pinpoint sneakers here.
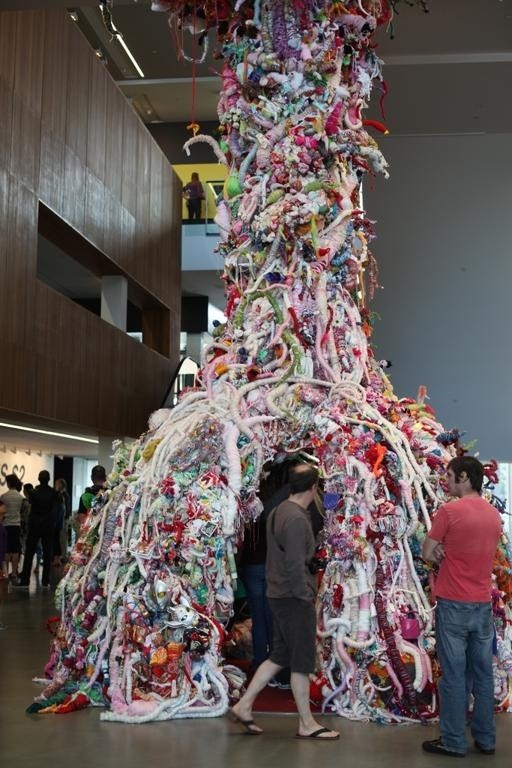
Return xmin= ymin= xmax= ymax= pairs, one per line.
xmin=12 ymin=582 xmax=30 ymax=588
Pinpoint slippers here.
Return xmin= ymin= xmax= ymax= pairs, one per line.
xmin=295 ymin=728 xmax=340 ymax=740
xmin=228 ymin=709 xmax=262 ymax=733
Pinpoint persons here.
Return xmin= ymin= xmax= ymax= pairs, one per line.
xmin=239 ymin=466 xmax=291 ymax=690
xmin=424 ymin=457 xmax=504 ymax=755
xmin=0 ymin=465 xmax=105 ymax=588
xmin=224 ymin=463 xmax=343 ymax=741
xmin=183 ymin=172 xmax=207 ymax=219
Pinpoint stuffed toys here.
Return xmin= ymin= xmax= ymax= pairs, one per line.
xmin=25 ymin=1 xmax=512 ymax=729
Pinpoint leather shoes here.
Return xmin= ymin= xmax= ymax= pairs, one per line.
xmin=474 ymin=739 xmax=495 ymax=755
xmin=422 ymin=737 xmax=464 ymax=757
